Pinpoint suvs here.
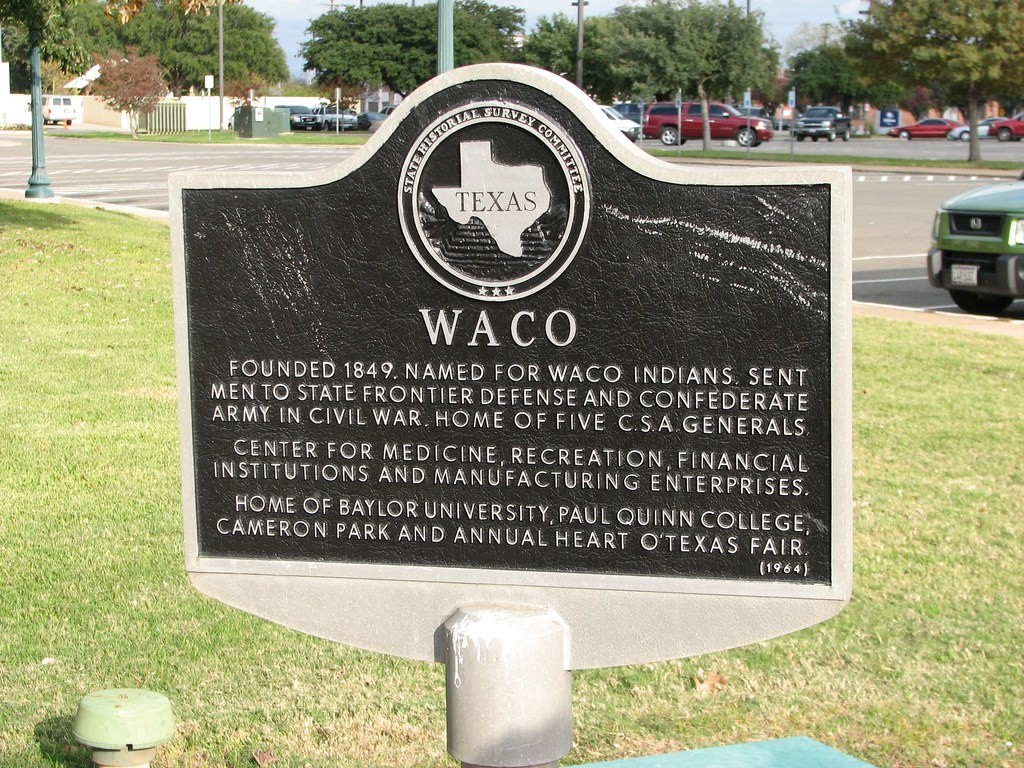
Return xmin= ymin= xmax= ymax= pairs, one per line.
xmin=272 ymin=105 xmax=317 ymax=131
xmin=310 ymin=106 xmax=358 ymax=131
xmin=793 ymin=106 xmax=851 ymax=142
xmin=643 ymin=102 xmax=774 ymax=147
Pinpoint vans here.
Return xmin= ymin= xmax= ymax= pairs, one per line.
xmin=27 ymin=94 xmax=76 ymax=126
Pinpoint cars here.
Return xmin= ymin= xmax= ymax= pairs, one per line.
xmin=598 ymin=105 xmax=642 ymax=143
xmin=988 ymin=111 xmax=1024 ymax=142
xmin=358 ymin=105 xmax=396 ymax=134
xmin=888 ymin=118 xmax=962 ymax=141
xmin=950 ymin=117 xmax=1010 ymax=142
xmin=925 ymin=170 xmax=1024 ymax=316
